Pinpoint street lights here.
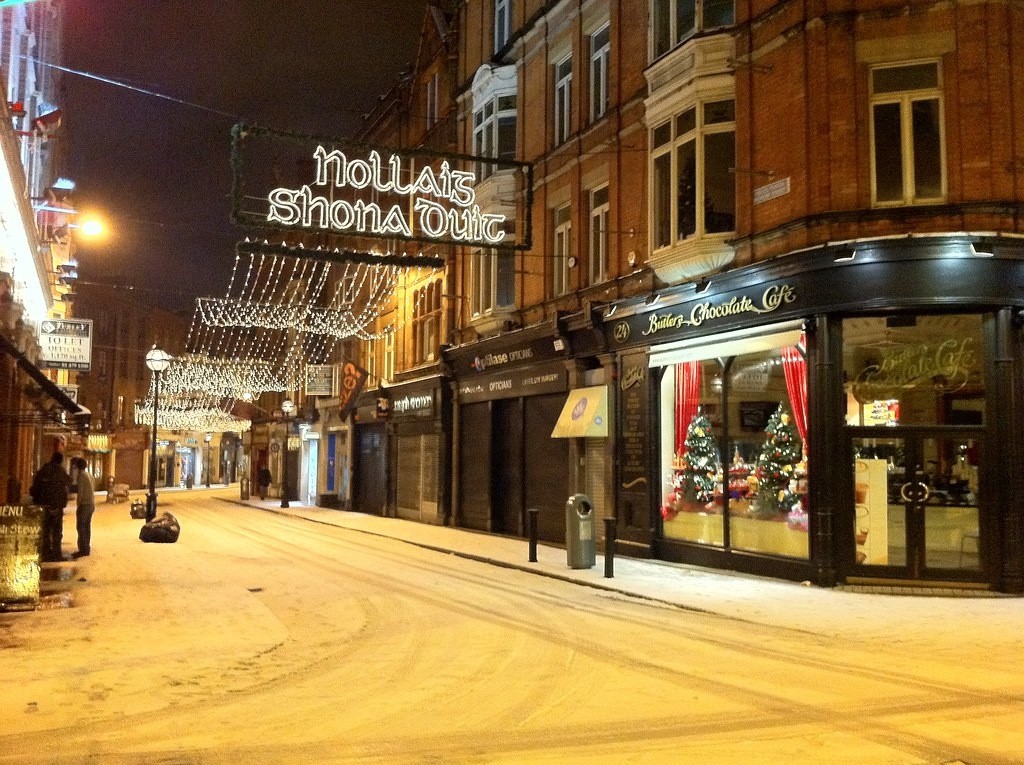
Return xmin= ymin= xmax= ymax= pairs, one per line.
xmin=279 ymin=400 xmax=294 ymax=508
xmin=205 ymin=433 xmax=213 ymax=488
xmin=145 ymin=347 xmax=171 ymax=523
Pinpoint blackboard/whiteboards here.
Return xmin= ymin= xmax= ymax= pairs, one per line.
xmin=0 ymin=504 xmax=44 ymax=605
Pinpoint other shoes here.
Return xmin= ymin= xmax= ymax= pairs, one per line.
xmin=71 ymin=550 xmax=90 ymax=556
xmin=43 ymin=554 xmax=68 ymax=561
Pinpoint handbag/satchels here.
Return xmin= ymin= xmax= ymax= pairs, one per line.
xmin=29 ymin=464 xmax=49 ymax=504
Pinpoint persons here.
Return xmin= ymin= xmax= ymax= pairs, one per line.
xmin=71 ymin=457 xmax=95 ymax=557
xmin=29 ymin=452 xmax=74 ymax=562
xmin=181 ymin=459 xmax=185 ymax=475
xmin=256 ymin=463 xmax=272 ymax=500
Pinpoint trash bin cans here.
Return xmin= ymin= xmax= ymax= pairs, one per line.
xmin=565 ymin=493 xmax=596 ymax=569
